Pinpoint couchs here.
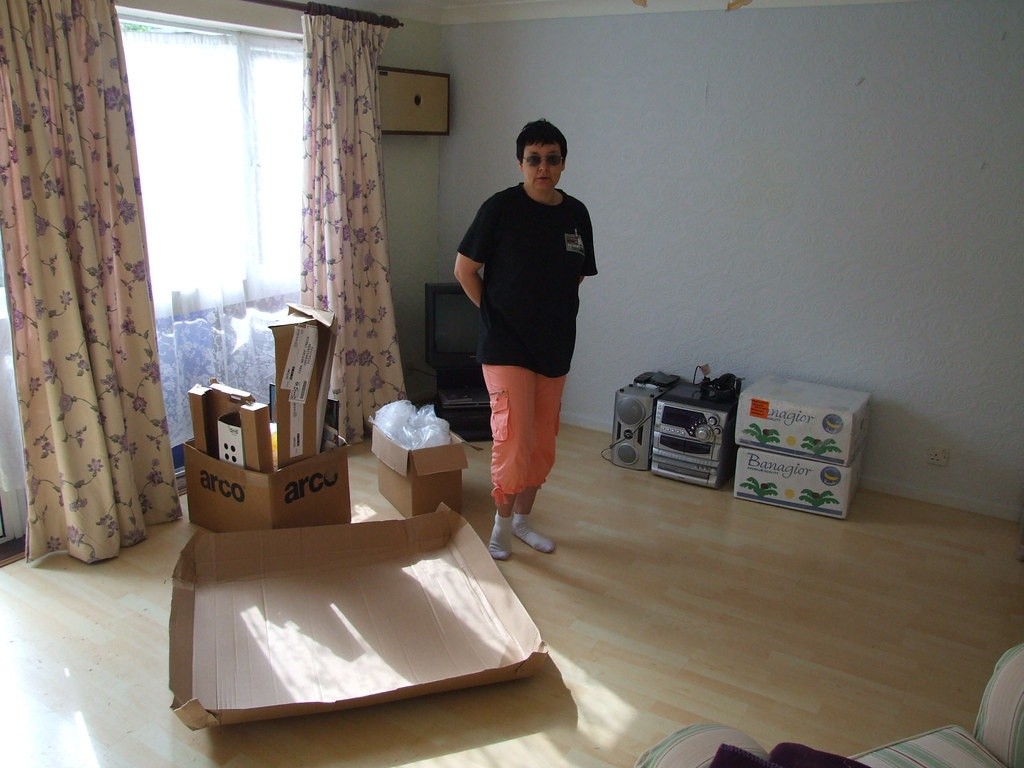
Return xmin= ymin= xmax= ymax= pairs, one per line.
xmin=634 ymin=642 xmax=1024 ymax=768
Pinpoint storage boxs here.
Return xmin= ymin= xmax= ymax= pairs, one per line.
xmin=733 ymin=447 xmax=863 ymax=519
xmin=183 ymin=305 xmax=485 ymax=534
xmin=735 ymin=379 xmax=871 ymax=467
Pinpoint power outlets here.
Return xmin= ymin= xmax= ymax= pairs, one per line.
xmin=929 ymin=449 xmax=947 ymax=466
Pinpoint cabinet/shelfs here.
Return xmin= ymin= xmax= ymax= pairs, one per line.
xmin=433 ymin=368 xmax=493 ymax=441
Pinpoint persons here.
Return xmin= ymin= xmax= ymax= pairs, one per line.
xmin=454 ymin=119 xmax=599 ymax=560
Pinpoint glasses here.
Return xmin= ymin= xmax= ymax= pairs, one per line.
xmin=523 ymin=155 xmax=562 ymax=167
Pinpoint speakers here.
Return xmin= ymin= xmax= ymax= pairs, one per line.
xmin=611 ymin=381 xmax=682 ymax=471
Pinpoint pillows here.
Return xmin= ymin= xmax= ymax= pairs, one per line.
xmin=769 ymin=742 xmax=870 ymax=768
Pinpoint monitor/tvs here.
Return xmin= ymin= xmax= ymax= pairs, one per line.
xmin=424 ymin=282 xmax=486 ymax=369
